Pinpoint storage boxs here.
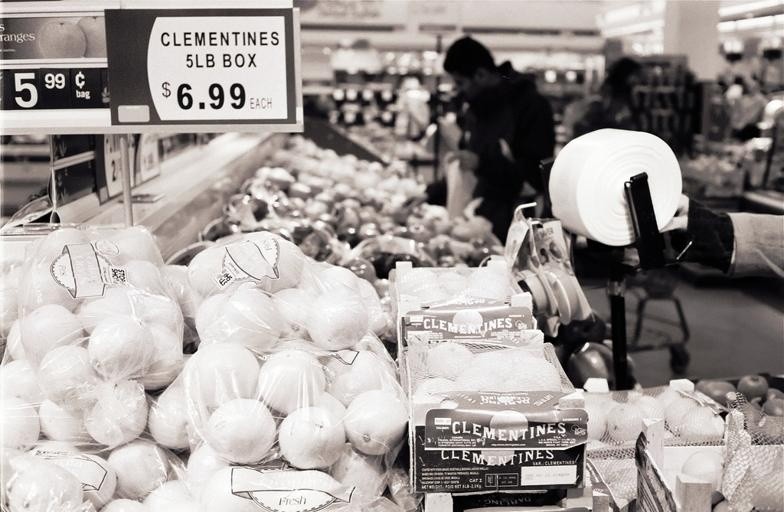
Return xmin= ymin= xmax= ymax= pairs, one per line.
xmin=397 ymin=315 xmax=589 ymax=496
xmin=636 ymin=444 xmax=783 ymax=511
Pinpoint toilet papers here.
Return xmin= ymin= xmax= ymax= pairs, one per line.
xmin=546 ymin=126 xmax=683 ymax=248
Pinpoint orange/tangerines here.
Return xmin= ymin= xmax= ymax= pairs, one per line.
xmin=0 ymin=228 xmax=409 ymax=512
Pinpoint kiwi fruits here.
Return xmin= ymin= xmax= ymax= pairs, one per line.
xmin=706 ymin=372 xmax=783 ymax=437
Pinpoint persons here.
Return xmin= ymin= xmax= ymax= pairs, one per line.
xmin=731 ymin=71 xmax=767 ymax=139
xmin=439 ymin=38 xmax=554 ymax=244
xmin=573 ymin=58 xmax=641 ymax=137
xmin=395 ymin=77 xmax=431 ymax=139
